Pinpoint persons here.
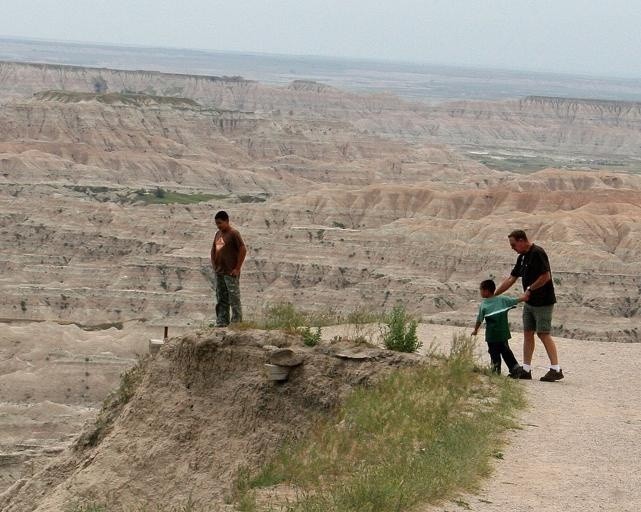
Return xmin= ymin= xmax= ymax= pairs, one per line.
xmin=211 ymin=211 xmax=247 ymax=328
xmin=470 ymin=279 xmax=528 ymax=375
xmin=494 ymin=229 xmax=564 ymax=381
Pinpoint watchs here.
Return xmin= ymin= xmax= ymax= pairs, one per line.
xmin=527 ymin=286 xmax=532 ymax=292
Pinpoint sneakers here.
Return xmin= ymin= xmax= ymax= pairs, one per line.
xmin=540 ymin=368 xmax=564 ymax=381
xmin=507 ymin=366 xmax=531 ymax=379
